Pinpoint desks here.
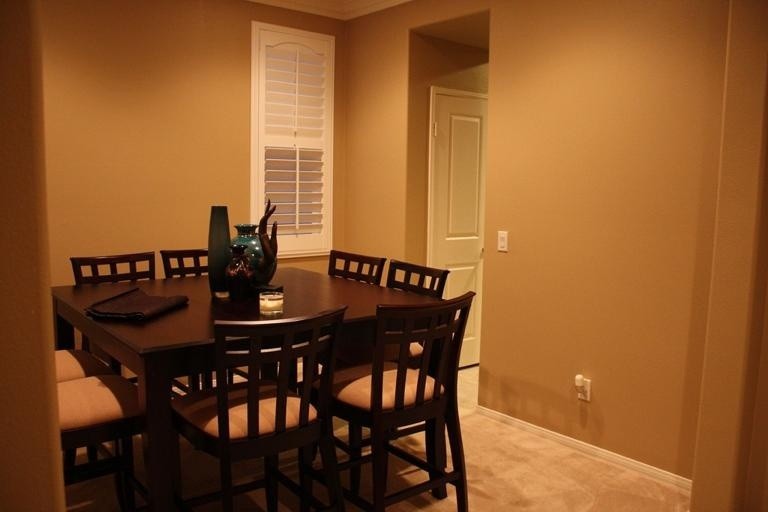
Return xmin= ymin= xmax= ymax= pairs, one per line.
xmin=51 ymin=265 xmax=450 ymax=512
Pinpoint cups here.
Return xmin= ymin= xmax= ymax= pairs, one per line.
xmin=258 ymin=291 xmax=285 ymax=317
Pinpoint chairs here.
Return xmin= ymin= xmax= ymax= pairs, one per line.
xmin=52 ymin=347 xmax=102 ymax=465
xmin=69 ymin=251 xmax=156 ymax=382
xmin=311 ymin=289 xmax=477 ymax=512
xmin=170 ymin=302 xmax=348 ymax=512
xmin=159 ymin=248 xmax=210 ymax=278
xmin=57 ymin=373 xmax=146 ymax=512
xmin=326 ymin=248 xmax=388 ymax=287
xmin=373 ymin=257 xmax=450 ymax=364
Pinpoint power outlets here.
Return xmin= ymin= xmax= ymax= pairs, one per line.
xmin=577 ymin=378 xmax=591 ymax=402
